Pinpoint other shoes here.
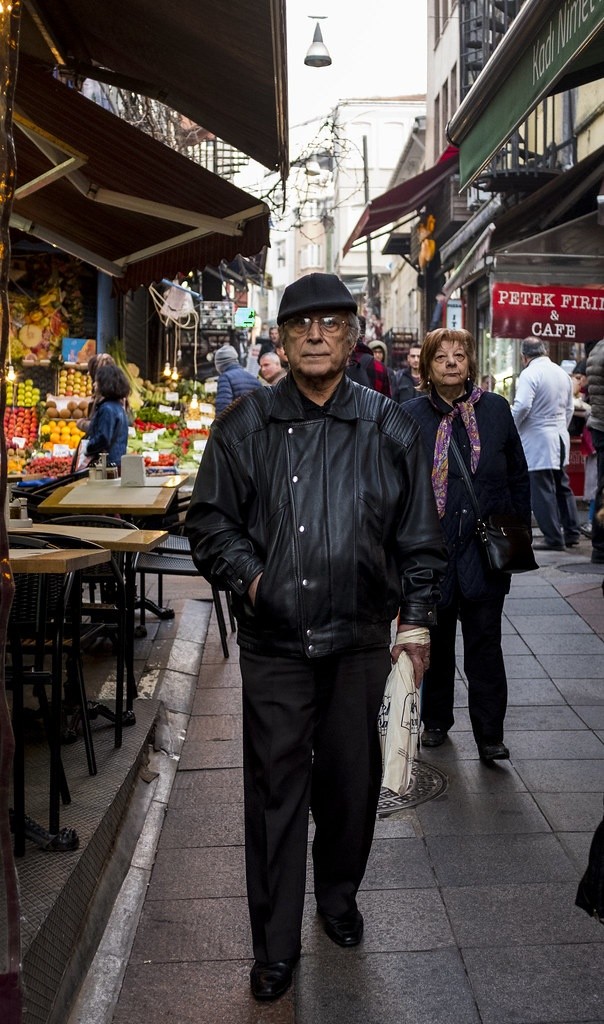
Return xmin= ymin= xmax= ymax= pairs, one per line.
xmin=564 ymin=538 xmax=579 ymax=548
xmin=533 ymin=538 xmax=566 ymax=551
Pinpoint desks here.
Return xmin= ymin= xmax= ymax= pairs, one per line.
xmin=7 ymin=523 xmax=168 ymax=710
xmin=8 ymin=548 xmax=111 ymax=805
xmin=36 ymin=475 xmax=191 ymax=618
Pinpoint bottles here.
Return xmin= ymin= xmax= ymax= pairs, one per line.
xmin=10 ymin=498 xmax=28 ymax=519
xmin=90 ymin=463 xmax=118 ymax=479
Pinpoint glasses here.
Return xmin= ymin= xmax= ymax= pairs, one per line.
xmin=280 ymin=315 xmax=351 ymax=334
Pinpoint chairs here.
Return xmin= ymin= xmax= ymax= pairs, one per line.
xmin=5 ymin=496 xmax=238 ymax=858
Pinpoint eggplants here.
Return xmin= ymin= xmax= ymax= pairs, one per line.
xmin=142 ymin=399 xmax=188 ymax=413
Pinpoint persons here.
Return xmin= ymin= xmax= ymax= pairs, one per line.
xmin=481 ymin=375 xmax=496 ymax=392
xmin=184 ymin=273 xmax=449 ymax=1003
xmin=391 ymin=344 xmax=423 ymax=386
xmin=257 ymin=323 xmax=395 ymax=400
xmin=509 ymin=336 xmax=581 ymax=550
xmin=568 ymin=339 xmax=604 ymax=564
xmin=214 ymin=345 xmax=262 ymax=416
xmin=399 ymin=327 xmax=540 ymax=765
xmin=76 ymin=353 xmax=133 ymax=477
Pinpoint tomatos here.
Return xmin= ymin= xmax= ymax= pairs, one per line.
xmin=144 ymin=453 xmax=179 ymax=467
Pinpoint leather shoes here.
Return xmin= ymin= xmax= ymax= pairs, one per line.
xmin=322 ymin=908 xmax=363 ymax=945
xmin=251 ymin=952 xmax=300 ymax=997
xmin=480 ymin=742 xmax=509 ymax=760
xmin=421 ymin=726 xmax=448 ymax=745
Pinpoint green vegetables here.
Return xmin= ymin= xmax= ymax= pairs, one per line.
xmin=127 ymin=406 xmax=199 ymax=467
xmin=175 ymin=379 xmax=217 ymax=405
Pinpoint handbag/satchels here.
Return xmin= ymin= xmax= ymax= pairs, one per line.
xmin=478 ymin=521 xmax=536 ymax=567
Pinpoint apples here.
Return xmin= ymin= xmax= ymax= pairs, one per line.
xmin=4 ymin=378 xmax=41 ymax=448
xmin=59 ymin=368 xmax=93 ymax=397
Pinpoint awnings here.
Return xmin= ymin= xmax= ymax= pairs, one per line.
xmin=445 ymin=0 xmax=604 ymax=193
xmin=343 ymin=145 xmax=604 ymax=296
xmin=8 ymin=61 xmax=271 ymax=302
xmin=0 ymin=0 xmax=291 ymax=213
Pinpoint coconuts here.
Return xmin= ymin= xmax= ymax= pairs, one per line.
xmin=45 ymin=400 xmax=90 ymax=419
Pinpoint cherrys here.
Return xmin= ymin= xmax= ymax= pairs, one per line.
xmin=25 ymin=455 xmax=75 ymax=477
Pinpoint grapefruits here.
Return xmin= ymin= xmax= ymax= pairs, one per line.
xmin=40 ymin=420 xmax=86 ymax=449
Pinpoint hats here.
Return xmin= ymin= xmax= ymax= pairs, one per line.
xmin=214 ymin=345 xmax=238 ymax=370
xmin=277 ymin=273 xmax=358 ymax=324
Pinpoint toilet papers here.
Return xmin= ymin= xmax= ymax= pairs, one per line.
xmin=121 ymin=455 xmax=148 ymax=487
